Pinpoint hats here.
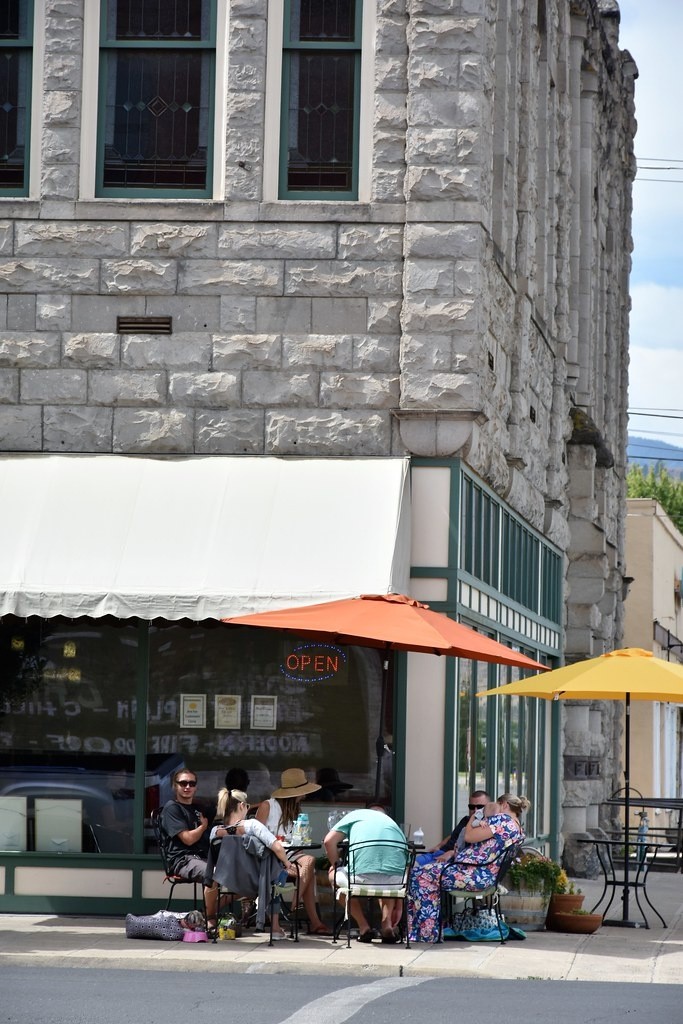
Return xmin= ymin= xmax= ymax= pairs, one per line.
xmin=271 ymin=768 xmax=322 ymax=798
xmin=315 ymin=768 xmax=353 ymax=791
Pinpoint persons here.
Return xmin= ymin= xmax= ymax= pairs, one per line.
xmin=255 ymin=768 xmax=335 ymax=936
xmin=322 ymin=796 xmax=406 ymax=944
xmin=80 ymin=770 xmax=133 ymax=854
xmin=406 ymin=794 xmax=536 ymax=943
xmin=220 ymin=786 xmax=298 ymax=941
xmin=157 ymin=769 xmax=243 ymax=939
xmin=470 ymin=798 xmax=500 ymax=828
xmin=409 ymin=791 xmax=491 ymax=867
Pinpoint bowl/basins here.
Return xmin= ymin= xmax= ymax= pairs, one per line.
xmin=183 ymin=932 xmax=208 ymax=942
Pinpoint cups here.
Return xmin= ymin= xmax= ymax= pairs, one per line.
xmin=400 ymin=824 xmax=411 ymax=840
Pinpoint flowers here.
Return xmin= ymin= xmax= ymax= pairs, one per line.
xmin=505 ymin=852 xmax=562 ymax=912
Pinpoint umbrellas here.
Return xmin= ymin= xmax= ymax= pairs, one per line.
xmin=474 ymin=647 xmax=683 ymax=924
xmin=220 ymin=591 xmax=550 ymax=803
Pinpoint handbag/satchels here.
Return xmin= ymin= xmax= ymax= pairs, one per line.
xmin=126 ymin=911 xmax=185 ymax=941
xmin=453 ymin=898 xmax=503 ymax=933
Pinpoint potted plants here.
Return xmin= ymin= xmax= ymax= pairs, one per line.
xmin=556 ymin=909 xmax=602 ymax=934
xmin=547 ymin=870 xmax=585 ymax=930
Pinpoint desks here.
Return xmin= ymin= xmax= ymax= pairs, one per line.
xmin=574 ymin=838 xmax=676 ymax=929
xmin=282 ymin=840 xmax=425 ymax=940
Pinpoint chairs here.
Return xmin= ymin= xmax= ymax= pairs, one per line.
xmin=149 ymin=807 xmax=525 ymax=942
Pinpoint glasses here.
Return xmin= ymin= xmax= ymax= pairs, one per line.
xmin=468 ymin=804 xmax=485 ymax=810
xmin=175 ymin=780 xmax=196 ymax=787
xmin=244 ymin=804 xmax=250 ymax=809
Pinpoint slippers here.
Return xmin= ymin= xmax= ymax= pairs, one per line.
xmin=307 ymin=924 xmax=334 ymax=935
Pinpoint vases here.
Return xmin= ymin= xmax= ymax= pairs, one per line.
xmin=498 ymin=873 xmax=553 ymax=932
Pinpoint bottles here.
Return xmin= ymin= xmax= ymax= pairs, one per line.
xmin=413 ymin=827 xmax=424 ymax=846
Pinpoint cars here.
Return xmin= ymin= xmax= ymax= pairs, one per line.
xmin=1 ymin=752 xmax=183 ymax=851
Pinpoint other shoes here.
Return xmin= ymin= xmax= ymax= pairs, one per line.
xmin=206 ymin=916 xmax=218 ymax=938
xmin=357 ymin=931 xmax=372 ymax=942
xmin=382 ymin=926 xmax=401 ymax=944
xmin=271 ymin=929 xmax=286 ymax=940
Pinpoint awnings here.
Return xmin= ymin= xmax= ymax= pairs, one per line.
xmin=0 ymin=452 xmax=414 ymax=621
